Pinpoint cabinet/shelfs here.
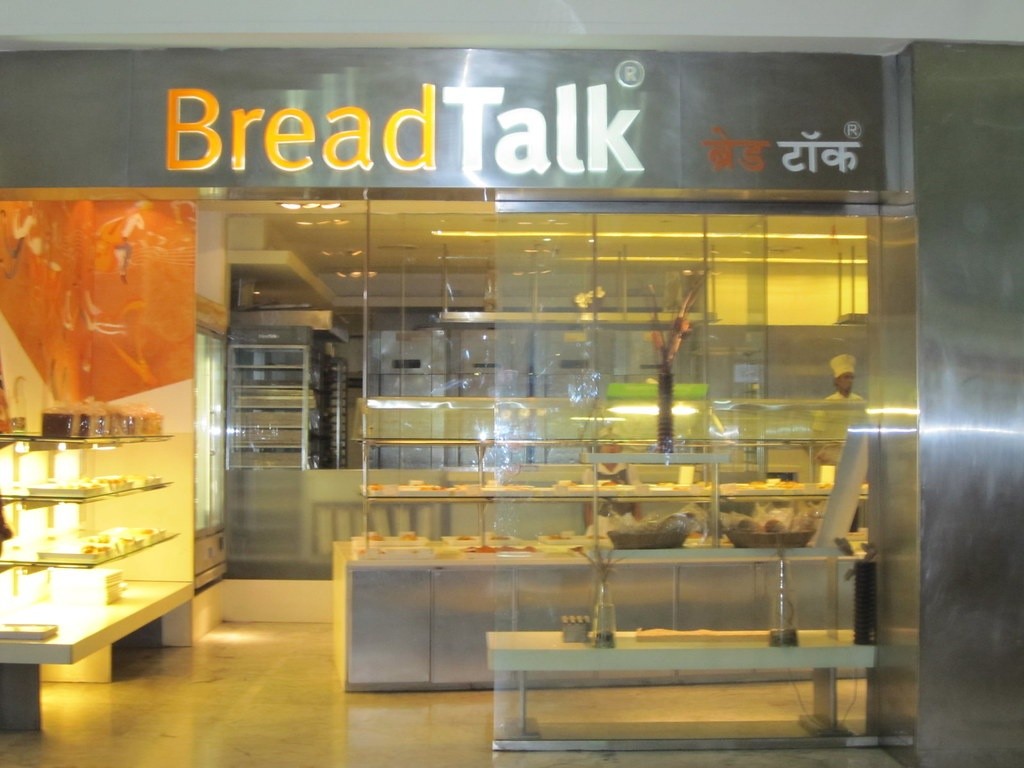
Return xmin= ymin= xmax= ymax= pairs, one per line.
xmin=355 ymin=394 xmax=869 ymax=546
xmin=331 ymin=534 xmax=868 ymax=687
xmin=0 ymin=427 xmax=193 ymax=662
xmin=224 ymin=342 xmax=323 ymax=470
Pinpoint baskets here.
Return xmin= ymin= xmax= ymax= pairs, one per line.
xmin=725 ymin=526 xmax=817 ymax=548
xmin=607 ymin=513 xmax=691 ymax=549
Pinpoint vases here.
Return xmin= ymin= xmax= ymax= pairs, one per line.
xmin=770 ymin=561 xmax=799 ymax=646
xmin=624 ymin=464 xmax=695 ymax=520
xmin=595 ymin=584 xmax=617 ymax=648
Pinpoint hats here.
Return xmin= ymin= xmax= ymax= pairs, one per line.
xmin=831 ymin=354 xmax=858 ymax=380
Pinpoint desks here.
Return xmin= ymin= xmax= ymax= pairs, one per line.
xmin=484 ymin=631 xmax=878 ymax=750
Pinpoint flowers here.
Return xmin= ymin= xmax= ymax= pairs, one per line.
xmin=775 ymin=535 xmax=786 ymax=559
xmin=637 ymin=269 xmax=705 ymax=463
xmin=573 ymin=543 xmax=625 ymax=580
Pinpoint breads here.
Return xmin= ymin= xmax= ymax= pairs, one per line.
xmin=42 ymin=411 xmax=164 ymax=438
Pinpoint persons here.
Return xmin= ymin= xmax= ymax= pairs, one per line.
xmin=585 ymin=436 xmax=643 ymax=539
xmin=812 ymin=353 xmax=868 ymax=532
